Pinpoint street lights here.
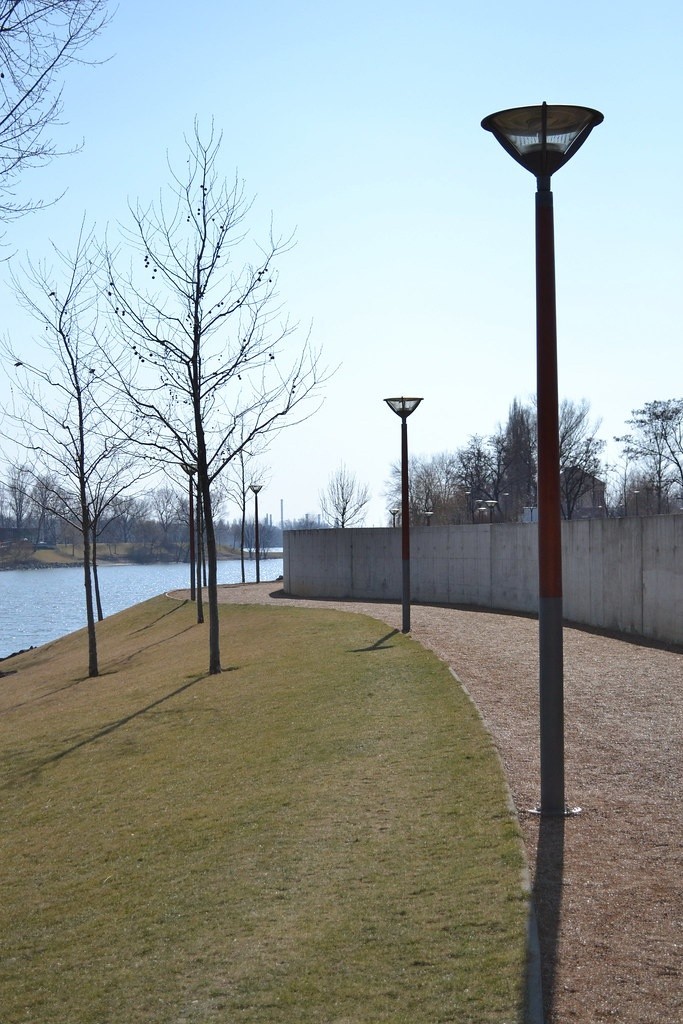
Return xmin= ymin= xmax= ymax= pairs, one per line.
xmin=478 ymin=508 xmax=486 ymax=523
xmin=180 ymin=463 xmax=199 ymax=600
xmin=425 ymin=512 xmax=434 ymax=526
xmin=479 ymin=101 xmax=607 ymax=819
xmin=383 ymin=396 xmax=424 ymax=631
xmin=389 ymin=509 xmax=399 ymax=527
xmin=484 ymin=500 xmax=498 ymax=524
xmin=248 ymin=484 xmax=265 ymax=583
